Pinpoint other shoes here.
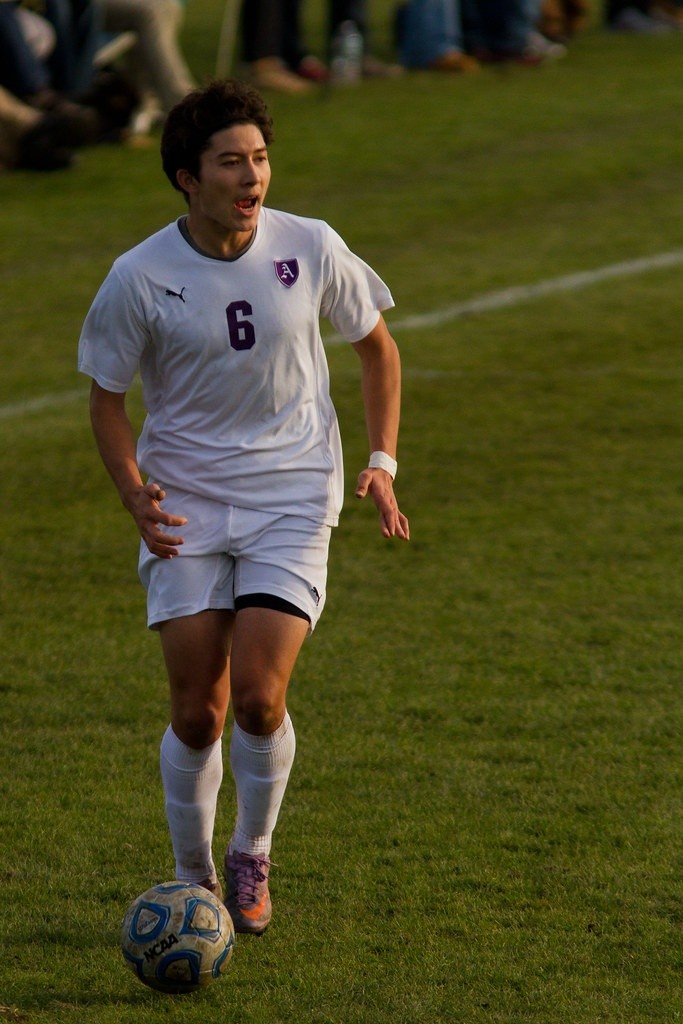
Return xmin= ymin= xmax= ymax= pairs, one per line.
xmin=429 ymin=48 xmax=479 ymax=73
xmin=615 ymin=8 xmax=672 ymax=32
xmin=476 ymin=33 xmax=562 ymax=67
xmin=362 ymin=54 xmax=404 ymax=78
xmin=296 ymin=53 xmax=334 ymax=84
xmin=253 ymin=58 xmax=311 ymax=97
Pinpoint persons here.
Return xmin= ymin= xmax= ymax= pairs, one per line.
xmin=0 ymin=0 xmax=683 ymax=168
xmin=77 ymin=77 xmax=411 ymax=931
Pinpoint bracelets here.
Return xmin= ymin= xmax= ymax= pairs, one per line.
xmin=368 ymin=451 xmax=398 ymax=480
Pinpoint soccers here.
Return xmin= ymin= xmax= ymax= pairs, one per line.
xmin=121 ymin=881 xmax=234 ymax=994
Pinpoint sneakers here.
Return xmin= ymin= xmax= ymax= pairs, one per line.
xmin=221 ymin=843 xmax=277 ymax=931
xmin=196 ymin=878 xmax=224 ymax=903
